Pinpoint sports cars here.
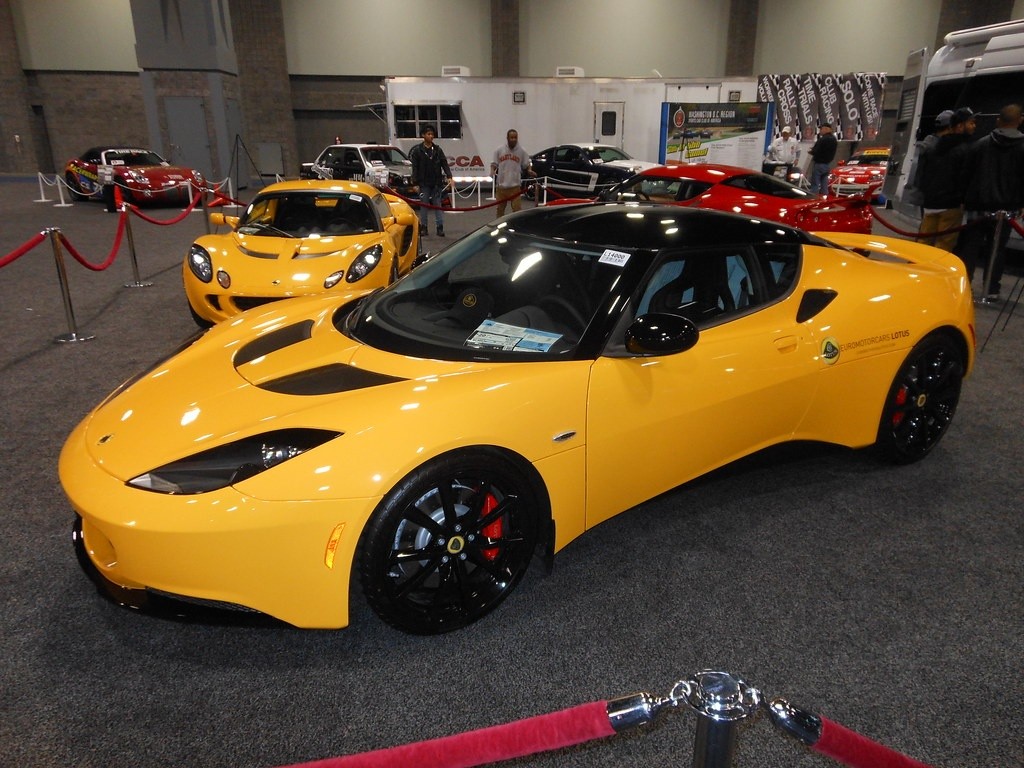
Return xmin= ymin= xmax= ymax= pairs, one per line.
xmin=541 ymin=162 xmax=874 ymax=258
xmin=59 ymin=203 xmax=977 ymax=639
xmin=65 ymin=145 xmax=207 ymax=212
xmin=182 ymin=179 xmax=424 ymax=329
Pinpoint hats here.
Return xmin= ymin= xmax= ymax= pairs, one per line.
xmin=950 ymin=107 xmax=981 ymax=127
xmin=935 ymin=110 xmax=954 ymax=126
xmin=818 ymin=123 xmax=831 ymax=128
xmin=782 ymin=126 xmax=791 ymax=133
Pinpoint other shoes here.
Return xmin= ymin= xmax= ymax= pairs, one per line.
xmin=436 ymin=224 xmax=445 ymax=236
xmin=421 ymin=225 xmax=428 ymax=235
xmin=987 ymin=294 xmax=999 ymax=302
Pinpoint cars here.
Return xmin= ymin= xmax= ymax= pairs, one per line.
xmin=302 ymin=136 xmax=451 ymax=211
xmin=827 ymin=145 xmax=896 ymax=206
xmin=520 ymin=141 xmax=665 ymax=202
xmin=673 ymin=129 xmax=713 ymax=139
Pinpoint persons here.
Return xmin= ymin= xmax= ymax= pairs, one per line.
xmin=911 ymin=104 xmax=1024 ymax=300
xmin=807 ymin=123 xmax=838 ymax=196
xmin=489 ymin=129 xmax=537 ymax=218
xmin=767 ymin=125 xmax=801 ymax=184
xmin=411 ymin=124 xmax=454 ymax=237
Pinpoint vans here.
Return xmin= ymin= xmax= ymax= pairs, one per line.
xmin=880 ymin=20 xmax=1024 ymax=253
